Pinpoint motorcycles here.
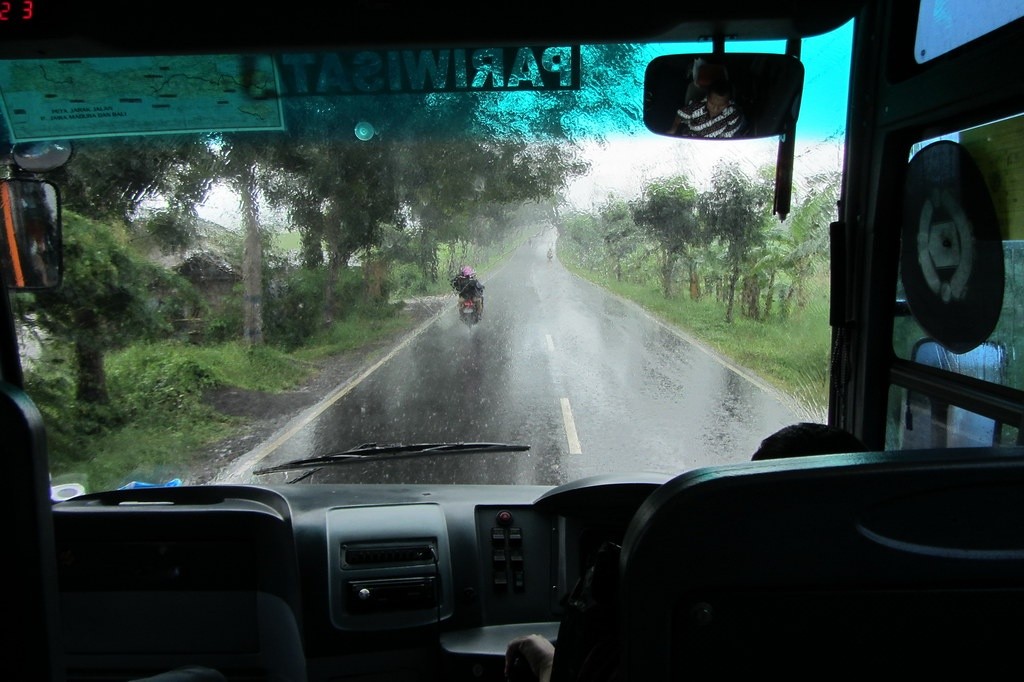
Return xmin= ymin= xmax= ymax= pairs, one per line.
xmin=461 ymin=297 xmax=477 ymax=329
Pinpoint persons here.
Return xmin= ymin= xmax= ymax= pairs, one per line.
xmin=503 ymin=423 xmax=893 ymax=682
xmin=667 ymin=84 xmax=746 ymax=140
xmin=457 ymin=267 xmax=485 ymax=320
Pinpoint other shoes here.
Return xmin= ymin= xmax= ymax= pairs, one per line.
xmin=477 ymin=314 xmax=482 ymax=320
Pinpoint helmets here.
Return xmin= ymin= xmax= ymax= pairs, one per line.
xmin=462 ymin=267 xmax=472 ymax=275
